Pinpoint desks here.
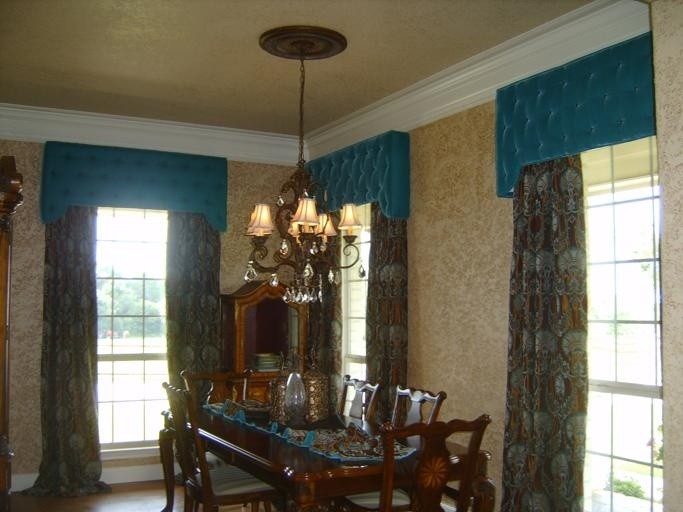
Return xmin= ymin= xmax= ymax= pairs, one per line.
xmin=159 ymin=408 xmax=496 ymax=512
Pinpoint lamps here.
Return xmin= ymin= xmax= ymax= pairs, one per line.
xmin=243 ymin=25 xmax=365 ymax=305
